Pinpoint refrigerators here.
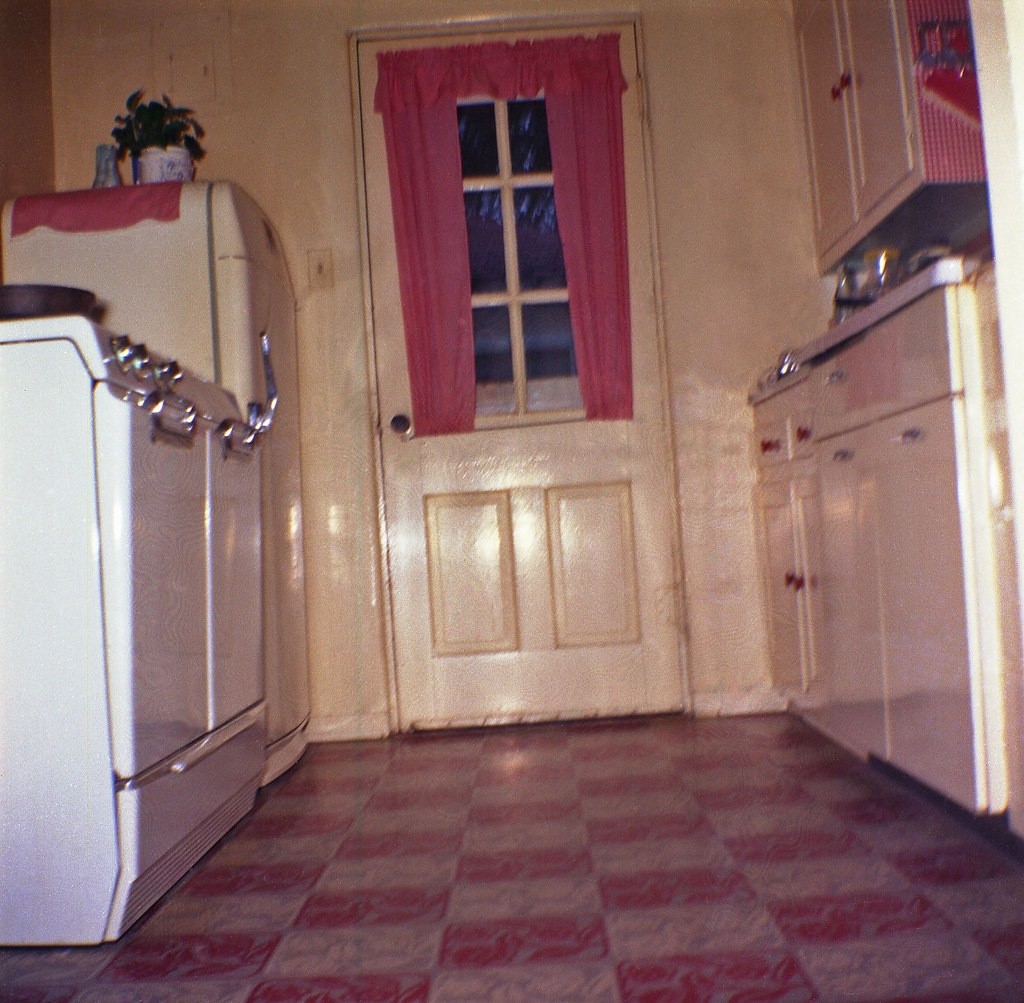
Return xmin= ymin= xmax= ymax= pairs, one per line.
xmin=0 ymin=174 xmax=314 ymax=756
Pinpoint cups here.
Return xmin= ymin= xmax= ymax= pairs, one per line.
xmin=861 ymin=243 xmax=902 ymax=299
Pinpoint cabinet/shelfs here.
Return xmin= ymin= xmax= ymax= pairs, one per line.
xmin=790 ymin=0 xmax=993 ymax=275
xmin=750 ymin=264 xmax=1024 ymax=842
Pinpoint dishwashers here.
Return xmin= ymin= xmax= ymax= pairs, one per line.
xmin=88 ymin=378 xmax=269 ymax=780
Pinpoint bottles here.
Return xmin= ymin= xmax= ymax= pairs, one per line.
xmin=102 ymin=158 xmax=124 ymax=189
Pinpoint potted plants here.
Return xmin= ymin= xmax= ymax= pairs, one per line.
xmin=109 ymin=88 xmax=206 ymax=183
